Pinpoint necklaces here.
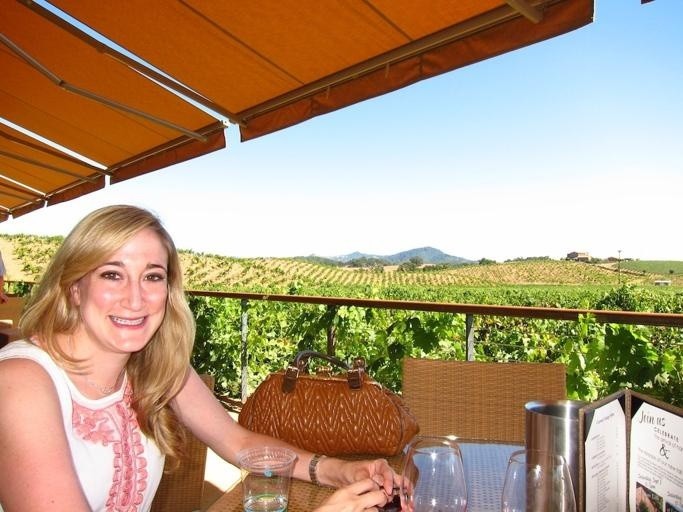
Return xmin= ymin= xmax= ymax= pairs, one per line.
xmin=66 ymin=333 xmax=116 ymax=394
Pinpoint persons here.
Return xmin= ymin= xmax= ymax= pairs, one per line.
xmin=0 ymin=204 xmax=415 ymax=512
xmin=0 ymin=250 xmax=9 ymax=305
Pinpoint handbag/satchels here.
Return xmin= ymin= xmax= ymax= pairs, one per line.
xmin=239 ymin=352 xmax=420 ymax=458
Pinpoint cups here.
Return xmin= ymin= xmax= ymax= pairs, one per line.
xmin=397 ymin=436 xmax=466 ymax=512
xmin=238 ymin=446 xmax=296 ymax=510
xmin=499 ymin=448 xmax=577 ymax=512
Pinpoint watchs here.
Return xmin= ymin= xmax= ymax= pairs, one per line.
xmin=308 ymin=452 xmax=327 ymax=487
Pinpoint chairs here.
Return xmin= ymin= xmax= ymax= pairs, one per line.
xmin=141 ymin=374 xmax=215 ymax=512
xmin=399 ymin=358 xmax=569 ymax=450
